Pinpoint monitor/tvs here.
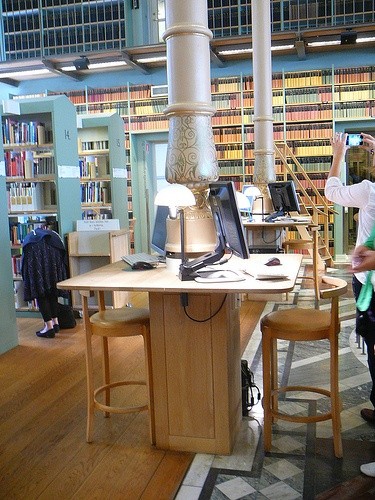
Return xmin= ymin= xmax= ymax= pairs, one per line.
xmin=149 ymin=203 xmax=171 ymax=263
xmin=264 ymin=181 xmax=300 ymax=223
xmin=179 ymin=180 xmax=250 ymax=280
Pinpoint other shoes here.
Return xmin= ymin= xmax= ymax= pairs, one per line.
xmin=36 ymin=328 xmax=56 ymax=338
xmin=52 ymin=324 xmax=59 ymax=333
xmin=359 ymin=462 xmax=375 ymax=477
xmin=359 ymin=408 xmax=375 ymax=422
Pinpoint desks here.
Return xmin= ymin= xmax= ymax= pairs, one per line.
xmin=244 ymin=222 xmax=311 ymax=253
xmin=57 ymin=253 xmax=303 ymax=455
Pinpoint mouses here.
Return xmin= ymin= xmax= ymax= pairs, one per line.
xmin=131 ymin=262 xmax=154 ymax=270
xmin=265 ymin=257 xmax=281 ymax=266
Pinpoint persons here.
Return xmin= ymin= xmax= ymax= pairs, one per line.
xmin=349 ymin=222 xmax=375 ymax=478
xmin=22 ymin=227 xmax=69 ymax=339
xmin=324 ymin=131 xmax=375 ymax=425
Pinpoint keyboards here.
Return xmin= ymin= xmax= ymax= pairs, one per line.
xmin=121 ymin=252 xmax=158 ymax=267
xmin=242 ymin=263 xmax=289 ymax=280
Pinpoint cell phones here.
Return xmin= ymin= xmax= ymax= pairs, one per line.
xmin=345 ymin=133 xmax=364 ymax=145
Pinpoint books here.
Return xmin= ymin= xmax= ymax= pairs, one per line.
xmin=2 ymin=117 xmax=56 ymax=309
xmin=210 ymin=68 xmax=333 ymax=258
xmin=79 ymin=141 xmax=112 ymax=219
xmin=125 ymin=133 xmax=135 ymax=256
xmin=334 ymin=66 xmax=375 ymax=119
xmin=49 ymin=90 xmax=86 ymax=115
xmin=12 ymin=93 xmax=46 ymax=100
xmin=87 ymin=84 xmax=169 ymax=131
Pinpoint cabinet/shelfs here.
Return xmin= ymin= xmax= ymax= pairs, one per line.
xmin=0 ymin=67 xmax=375 ymax=353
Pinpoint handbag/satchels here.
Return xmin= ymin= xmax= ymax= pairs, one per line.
xmin=57 ymin=304 xmax=77 ymax=328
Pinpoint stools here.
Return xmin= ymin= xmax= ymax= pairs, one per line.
xmin=260 ymin=275 xmax=347 ymax=459
xmin=283 ymin=224 xmax=321 ymax=302
xmin=78 ymin=290 xmax=155 ymax=448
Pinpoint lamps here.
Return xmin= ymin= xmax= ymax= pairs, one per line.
xmin=73 ymin=56 xmax=90 ymax=70
xmin=339 ymin=28 xmax=357 ymax=44
xmin=154 ymin=184 xmax=196 ymax=262
xmin=236 ymin=191 xmax=250 ymax=209
xmin=245 ymin=186 xmax=264 ymax=221
